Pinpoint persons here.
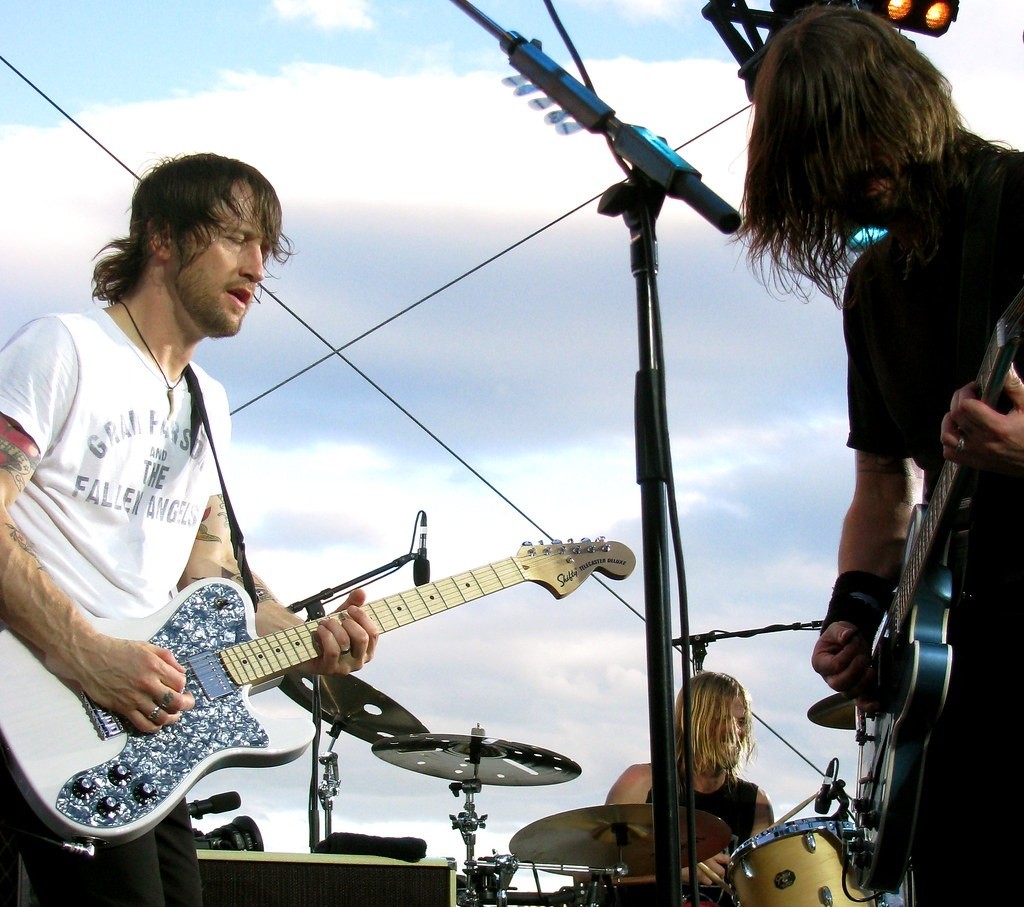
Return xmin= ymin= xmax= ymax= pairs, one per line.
xmin=742 ymin=9 xmax=1024 ymax=907
xmin=0 ymin=153 xmax=379 ymax=907
xmin=574 ymin=672 xmax=775 ymax=907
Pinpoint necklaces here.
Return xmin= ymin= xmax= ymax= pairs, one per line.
xmin=117 ymin=301 xmax=185 ymax=421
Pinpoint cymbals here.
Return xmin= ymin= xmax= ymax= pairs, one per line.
xmin=279 ymin=663 xmax=583 ymax=788
xmin=506 ymin=803 xmax=731 ymax=878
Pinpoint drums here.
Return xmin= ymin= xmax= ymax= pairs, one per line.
xmin=724 ymin=815 xmax=880 ymax=906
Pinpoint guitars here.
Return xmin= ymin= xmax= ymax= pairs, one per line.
xmin=0 ymin=533 xmax=638 ymax=850
xmin=843 ymin=286 xmax=1023 ymax=889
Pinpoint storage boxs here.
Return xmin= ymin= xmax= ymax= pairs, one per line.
xmin=195 ymin=849 xmax=457 ymax=907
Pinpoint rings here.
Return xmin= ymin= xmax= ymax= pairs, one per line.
xmin=955 ymin=438 xmax=964 ymax=454
xmin=341 ymin=647 xmax=350 ymax=655
xmin=147 ymin=706 xmax=162 ymax=720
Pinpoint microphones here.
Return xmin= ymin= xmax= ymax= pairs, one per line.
xmin=813 ymin=759 xmax=836 ymax=816
xmin=412 ymin=510 xmax=432 ymax=588
